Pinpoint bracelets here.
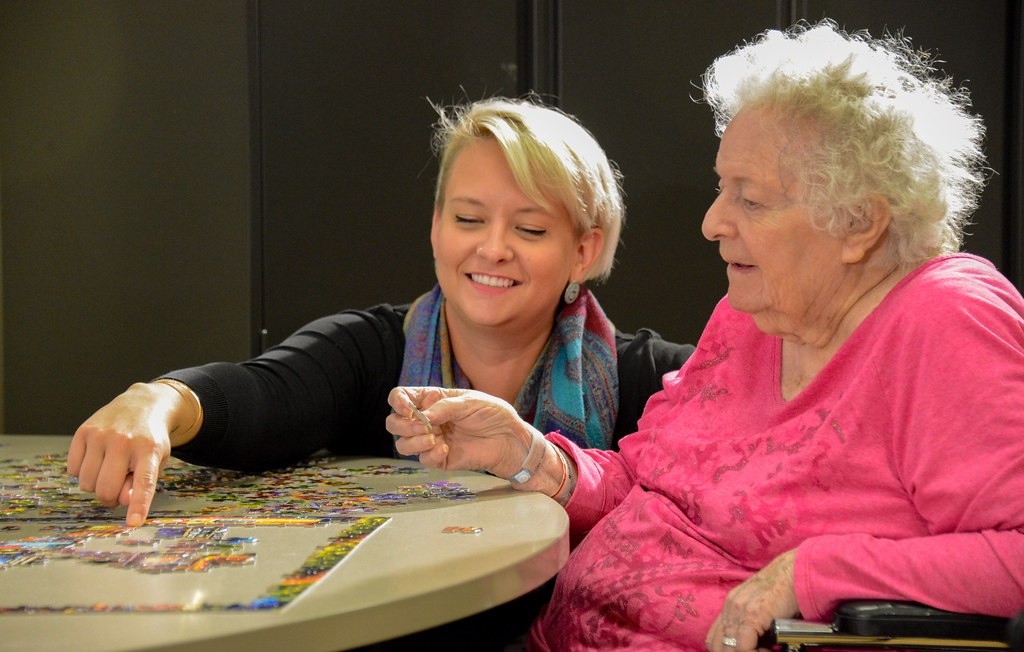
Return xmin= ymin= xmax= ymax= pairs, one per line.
xmin=510 ymin=429 xmax=545 ymax=482
xmin=548 ymin=441 xmax=578 ymax=508
xmin=151 ymin=379 xmax=201 ymax=435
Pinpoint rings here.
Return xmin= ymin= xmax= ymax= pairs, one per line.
xmin=722 ymin=638 xmax=736 ymax=646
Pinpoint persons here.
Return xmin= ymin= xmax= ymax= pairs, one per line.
xmin=385 ymin=25 xmax=1024 ymax=652
xmin=67 ymin=95 xmax=695 ymax=652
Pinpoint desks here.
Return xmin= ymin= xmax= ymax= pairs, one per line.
xmin=0 ymin=433 xmax=570 ymax=652
